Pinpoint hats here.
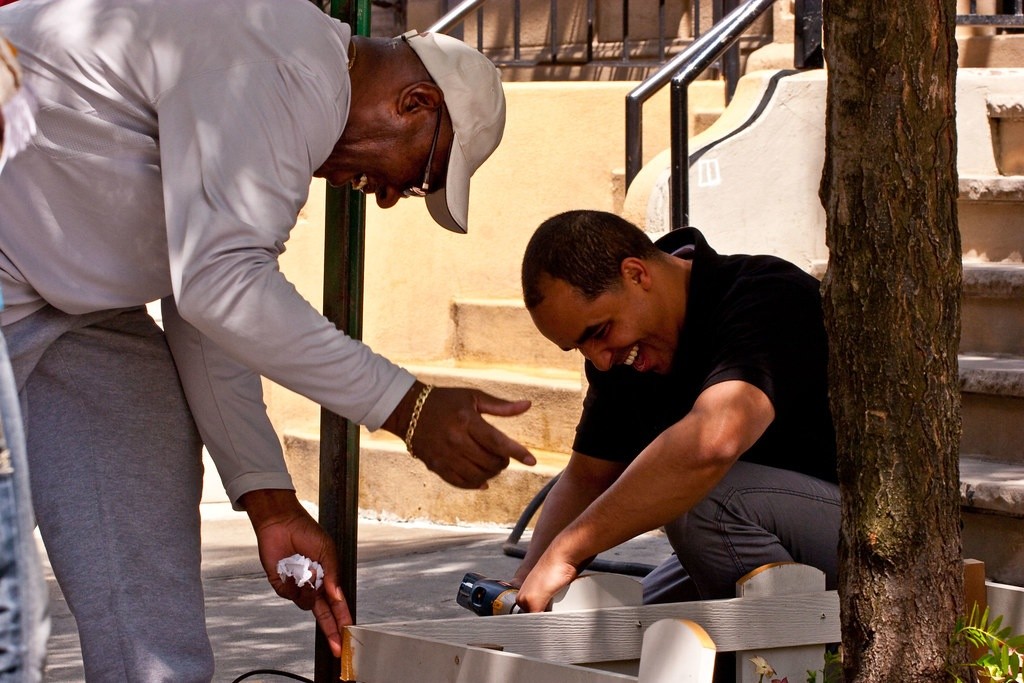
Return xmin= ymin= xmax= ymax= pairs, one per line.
xmin=394 ymin=27 xmax=506 ymax=234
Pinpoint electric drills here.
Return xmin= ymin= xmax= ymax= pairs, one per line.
xmin=455 ymin=572 xmax=525 ymax=616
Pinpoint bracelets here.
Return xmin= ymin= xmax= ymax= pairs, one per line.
xmin=406 ymin=383 xmax=434 ymax=459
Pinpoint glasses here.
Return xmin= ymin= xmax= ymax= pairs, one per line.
xmin=399 ymin=103 xmax=446 ymax=199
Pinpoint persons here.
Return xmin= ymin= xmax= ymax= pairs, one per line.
xmin=1 ymin=0 xmax=534 ymax=683
xmin=508 ymin=212 xmax=841 ymax=612
xmin=0 ymin=37 xmax=52 ymax=683
